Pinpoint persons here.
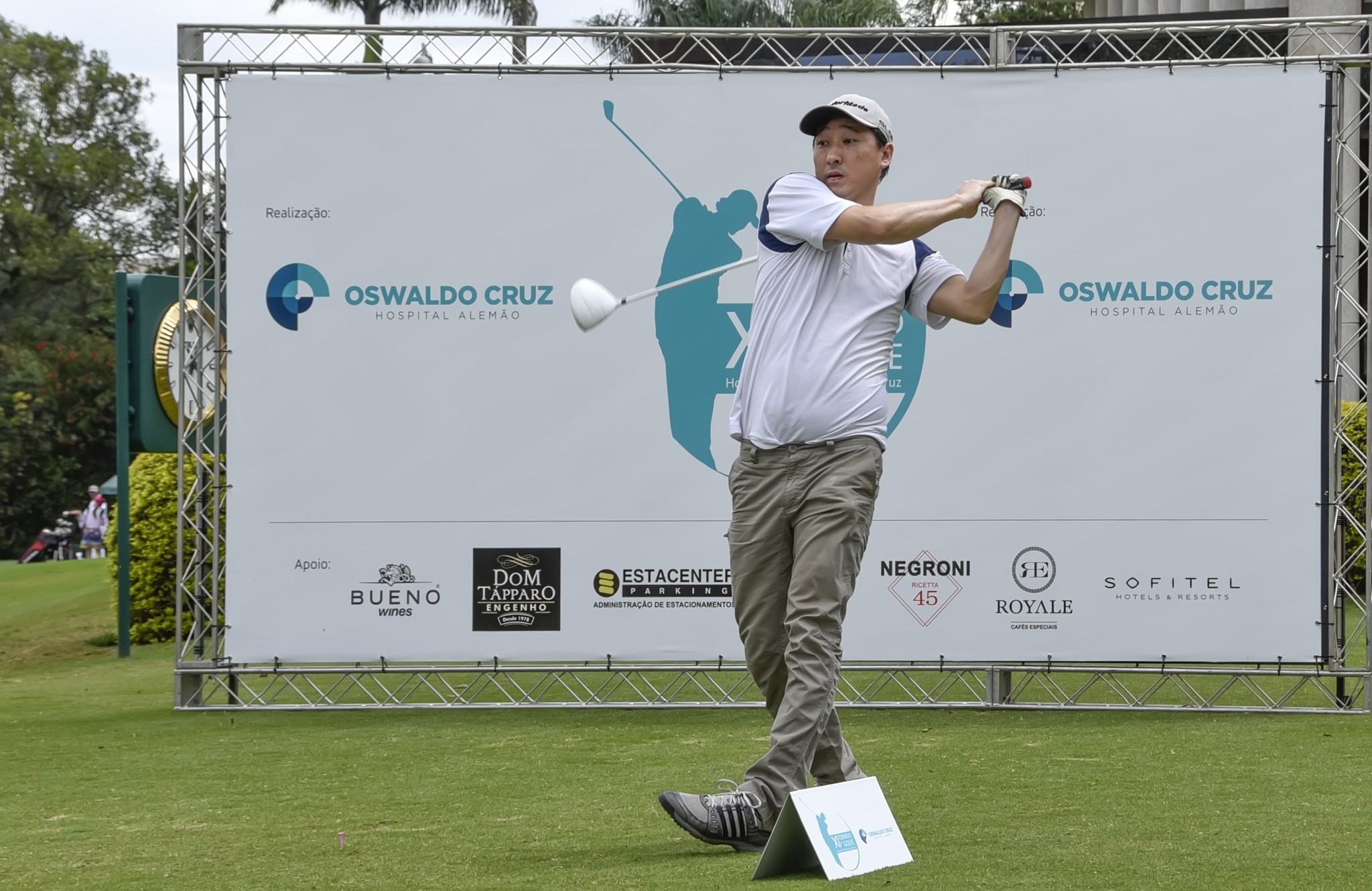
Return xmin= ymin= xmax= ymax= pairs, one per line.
xmin=659 ymin=92 xmax=1027 ymax=854
xmin=70 ymin=485 xmax=109 ymax=558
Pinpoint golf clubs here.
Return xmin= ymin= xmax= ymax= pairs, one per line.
xmin=568 ymin=175 xmax=1033 ymax=333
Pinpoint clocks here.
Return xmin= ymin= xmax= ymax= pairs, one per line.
xmin=155 ymin=300 xmax=229 ymax=428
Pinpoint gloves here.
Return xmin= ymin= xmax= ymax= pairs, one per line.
xmin=981 ymin=173 xmax=1026 ymax=218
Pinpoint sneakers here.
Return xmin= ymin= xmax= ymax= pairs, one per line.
xmin=659 ymin=779 xmax=771 ymax=854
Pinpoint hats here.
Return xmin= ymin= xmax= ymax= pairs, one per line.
xmin=88 ymin=484 xmax=100 ymax=493
xmin=94 ymin=494 xmax=105 ymax=501
xmin=798 ymin=94 xmax=893 ymax=145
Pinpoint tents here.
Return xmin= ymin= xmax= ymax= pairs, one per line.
xmin=100 ymin=475 xmax=117 ymax=496
